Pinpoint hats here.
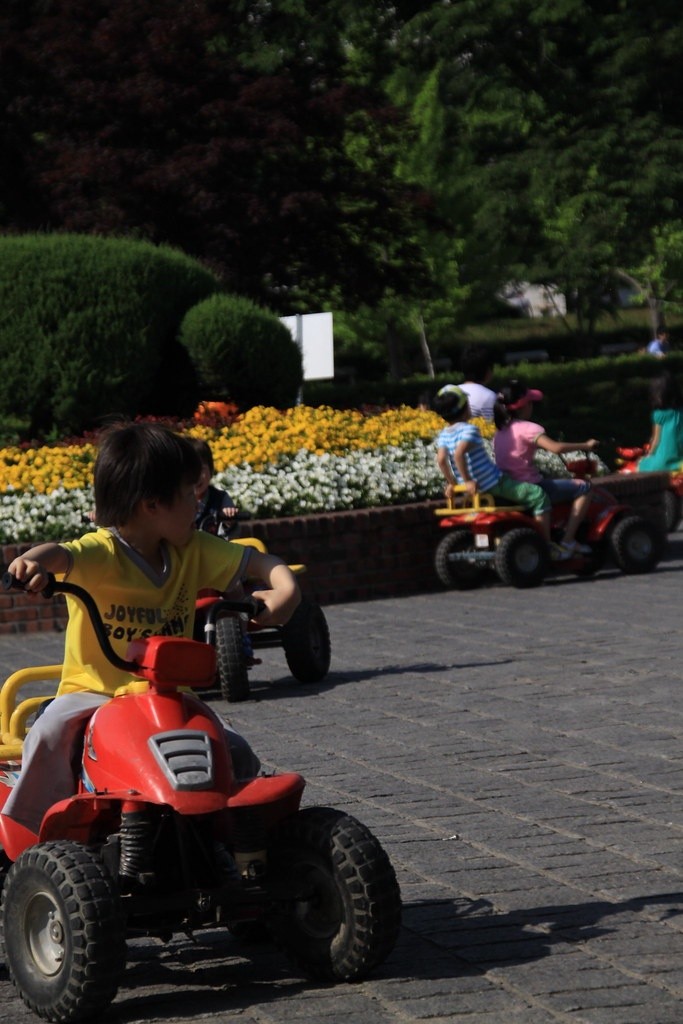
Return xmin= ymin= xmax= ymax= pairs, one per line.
xmin=431 ymin=384 xmax=470 ymax=419
xmin=500 ymin=386 xmax=544 ymax=411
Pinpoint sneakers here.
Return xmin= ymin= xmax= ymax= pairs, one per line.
xmin=547 ymin=542 xmax=572 ymax=561
xmin=559 ymin=540 xmax=592 ymax=554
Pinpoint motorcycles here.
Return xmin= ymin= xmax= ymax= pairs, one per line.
xmin=1 ymin=564 xmax=408 ymax=1023
xmin=190 ymin=506 xmax=332 ymax=703
xmin=609 ymin=444 xmax=683 ymax=532
xmin=430 ymin=440 xmax=672 ymax=589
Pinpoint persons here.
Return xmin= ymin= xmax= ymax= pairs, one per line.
xmin=0 ymin=423 xmax=301 ymax=871
xmin=646 ymin=325 xmax=670 ymax=358
xmin=493 ymin=385 xmax=600 ymax=554
xmin=637 ymin=387 xmax=683 ymax=473
xmin=458 ymin=358 xmax=497 ymax=420
xmin=181 ymin=435 xmax=253 ymax=669
xmin=434 ymin=385 xmax=573 ymax=561
